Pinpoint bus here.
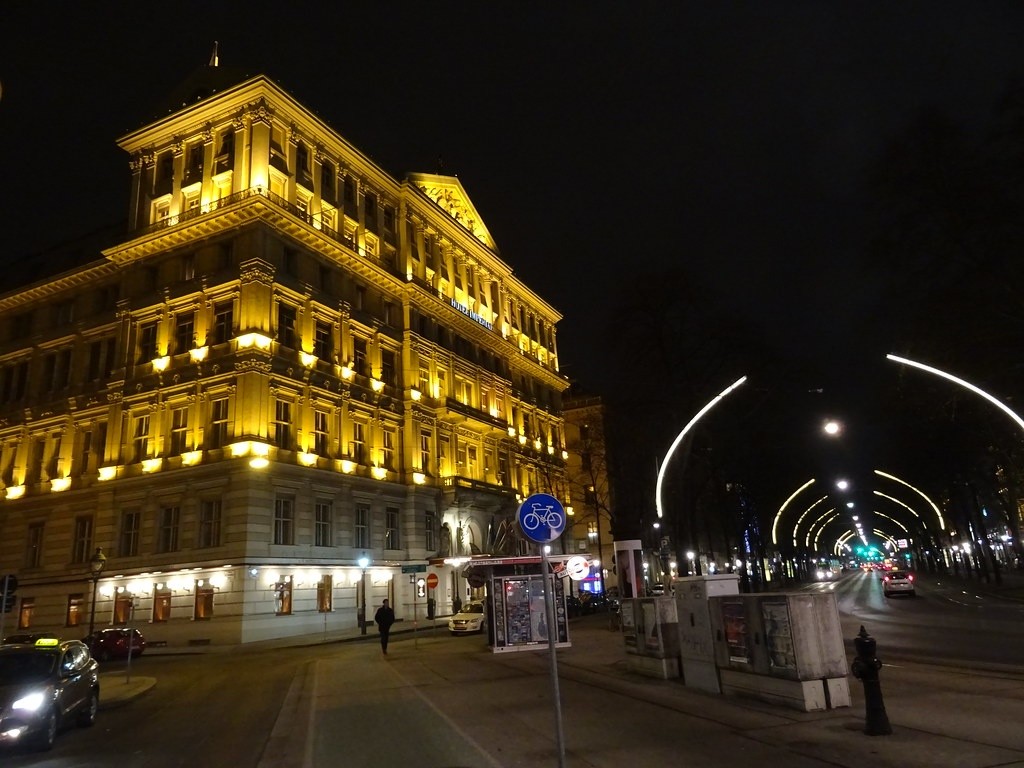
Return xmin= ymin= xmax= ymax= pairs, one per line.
xmin=816 ymin=558 xmax=842 ymax=581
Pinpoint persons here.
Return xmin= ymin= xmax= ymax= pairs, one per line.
xmin=454 ymin=597 xmax=461 ymax=614
xmin=375 ymin=599 xmax=395 ymax=655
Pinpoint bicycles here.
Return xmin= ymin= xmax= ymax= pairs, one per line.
xmin=567 ymin=588 xmax=621 ymax=632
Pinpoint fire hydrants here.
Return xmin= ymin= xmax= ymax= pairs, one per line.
xmin=850 ymin=624 xmax=893 ymax=736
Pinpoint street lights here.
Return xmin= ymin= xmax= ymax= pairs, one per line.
xmin=358 ymin=556 xmax=369 ymax=634
xmin=89 ymin=547 xmax=107 ymax=650
xmin=687 ymin=552 xmax=694 ymax=576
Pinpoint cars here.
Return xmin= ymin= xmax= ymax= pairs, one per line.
xmin=3 ymin=633 xmax=64 ymax=645
xmin=879 ymin=569 xmax=916 ymax=598
xmin=81 ymin=627 xmax=146 ymax=661
xmin=0 ymin=635 xmax=100 ymax=750
xmin=652 ymin=584 xmax=665 ymax=597
xmin=448 ymin=600 xmax=487 ymax=636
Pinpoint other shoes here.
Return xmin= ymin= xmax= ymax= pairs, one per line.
xmin=382 ymin=648 xmax=388 ymax=656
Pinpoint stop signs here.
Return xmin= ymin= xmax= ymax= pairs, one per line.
xmin=426 ymin=573 xmax=438 ymax=589
